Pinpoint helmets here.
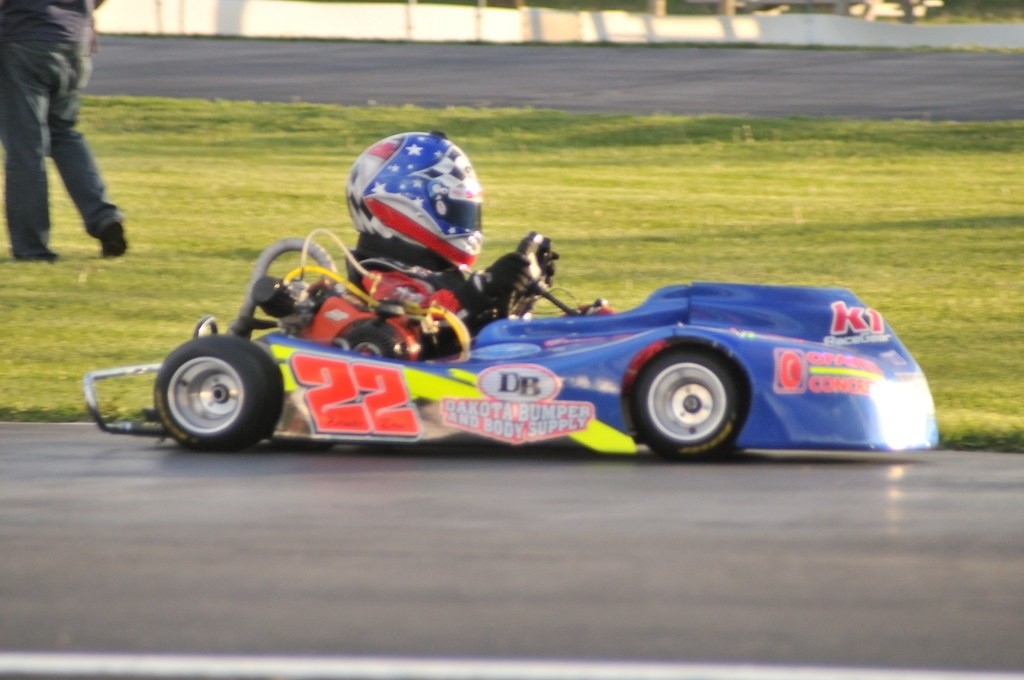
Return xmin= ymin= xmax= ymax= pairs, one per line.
xmin=347 ymin=131 xmax=483 ymax=274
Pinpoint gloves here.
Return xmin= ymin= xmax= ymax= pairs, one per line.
xmin=480 ymin=252 xmax=560 ymax=297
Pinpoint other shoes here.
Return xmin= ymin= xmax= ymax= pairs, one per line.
xmin=102 ymin=223 xmax=126 ymax=256
xmin=12 ymin=252 xmax=57 ymax=263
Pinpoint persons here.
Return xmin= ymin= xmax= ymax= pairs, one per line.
xmin=0 ymin=0 xmax=128 ymax=262
xmin=345 ymin=130 xmax=620 ymax=361
